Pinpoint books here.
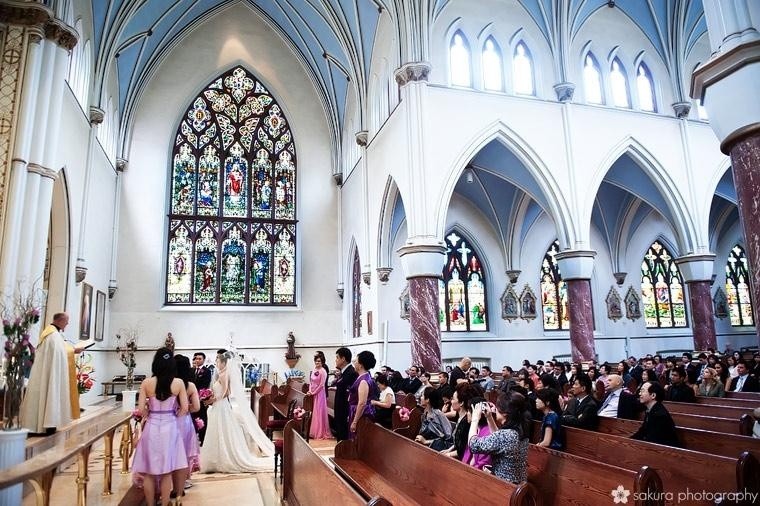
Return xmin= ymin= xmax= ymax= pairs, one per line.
xmin=74 ymin=338 xmax=94 ymax=349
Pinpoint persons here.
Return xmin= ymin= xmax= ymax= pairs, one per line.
xmin=165 ymin=332 xmax=174 ymax=353
xmin=130 ymin=346 xmax=189 ymax=505
xmin=19 ymin=312 xmax=86 ymax=436
xmin=286 ymin=331 xmax=298 ymax=360
xmin=189 ymin=348 xmax=252 ymax=475
xmin=306 ymin=346 xmax=760 ymax=485
xmin=168 ymin=354 xmax=201 ymax=505
xmin=178 ymin=162 xmax=294 ymax=210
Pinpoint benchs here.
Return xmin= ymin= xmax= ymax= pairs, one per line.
xmin=249 ymin=373 xmax=760 ymax=506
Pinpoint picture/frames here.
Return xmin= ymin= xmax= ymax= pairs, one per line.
xmin=499 ymin=282 xmax=519 ymax=322
xmin=400 ymin=285 xmax=410 ymax=319
xmin=713 ymin=285 xmax=728 ymax=320
xmin=624 ymin=285 xmax=641 ymax=321
xmin=605 ymin=284 xmax=623 ymax=322
xmin=520 ymin=282 xmax=537 ymax=322
xmin=80 ymin=281 xmax=93 ymax=340
xmin=94 ymin=289 xmax=106 ymax=341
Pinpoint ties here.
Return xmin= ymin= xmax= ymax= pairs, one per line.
xmin=734 ymin=379 xmax=743 ymax=391
xmin=194 ymin=367 xmax=200 ymax=375
xmin=599 ymin=393 xmax=614 ymax=412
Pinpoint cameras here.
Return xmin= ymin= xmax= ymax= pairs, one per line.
xmin=481 ymin=403 xmax=486 ymax=412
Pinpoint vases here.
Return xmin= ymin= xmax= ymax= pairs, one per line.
xmin=0 ymin=428 xmax=30 ymax=506
xmin=121 ymin=391 xmax=137 ymax=413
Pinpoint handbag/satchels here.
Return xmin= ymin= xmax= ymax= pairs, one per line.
xmin=418 ymin=422 xmax=444 ymax=439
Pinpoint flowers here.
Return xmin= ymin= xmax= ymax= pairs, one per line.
xmin=0 ymin=275 xmax=46 ymax=429
xmin=116 ymin=329 xmax=138 ymax=390
xmin=75 ymin=354 xmax=97 ymax=393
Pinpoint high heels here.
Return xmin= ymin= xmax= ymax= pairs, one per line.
xmin=176 ymin=496 xmax=183 ymax=506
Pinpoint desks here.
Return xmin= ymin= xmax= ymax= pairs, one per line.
xmin=101 ymin=382 xmax=146 ymax=396
